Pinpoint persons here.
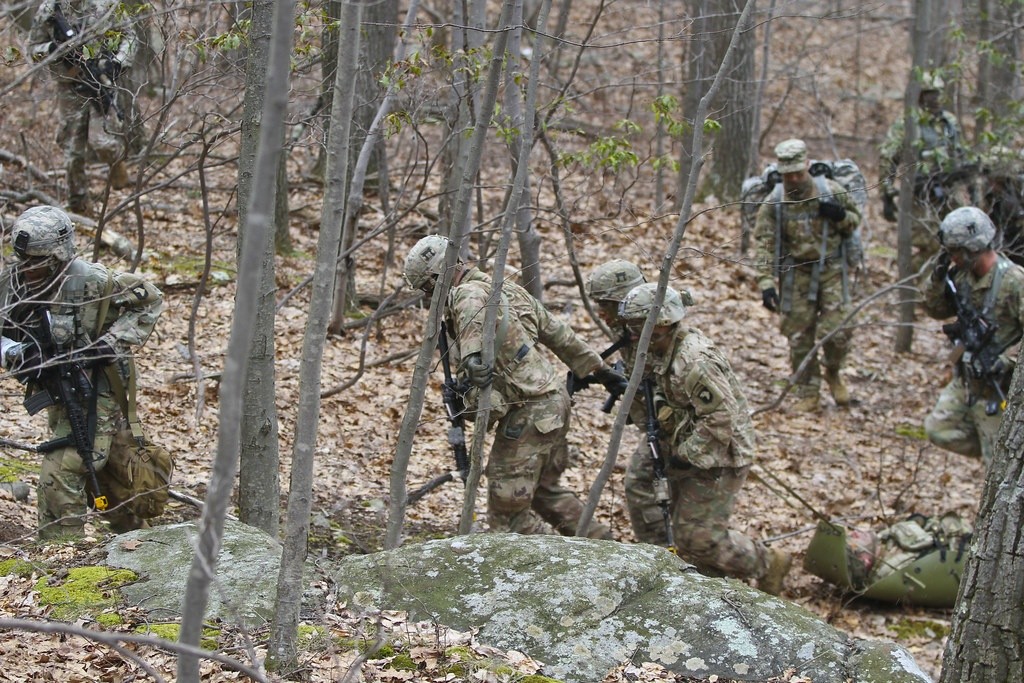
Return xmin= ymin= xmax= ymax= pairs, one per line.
xmin=916 ymin=207 xmax=1024 ymax=461
xmin=0 ymin=206 xmax=164 ymax=542
xmin=877 ymin=72 xmax=961 ymax=281
xmin=585 ymin=259 xmax=793 ymax=597
xmin=6 ymin=0 xmax=139 ymax=206
xmin=405 ymin=235 xmax=628 ymax=540
xmin=753 ymin=139 xmax=862 ymax=412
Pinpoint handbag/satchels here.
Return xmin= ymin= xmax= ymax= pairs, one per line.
xmin=102 ymin=429 xmax=174 ymax=519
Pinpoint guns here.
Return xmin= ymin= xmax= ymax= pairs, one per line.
xmin=437 ymin=319 xmax=470 ymax=490
xmin=16 ymin=304 xmax=110 ymax=514
xmin=942 ymin=273 xmax=1007 ymax=417
xmin=884 ymin=163 xmax=991 ymax=201
xmin=563 ymin=359 xmax=644 ymax=425
xmin=641 ymin=377 xmax=694 ymax=554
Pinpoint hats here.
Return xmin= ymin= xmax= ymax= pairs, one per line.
xmin=775 ymin=138 xmax=807 ymax=174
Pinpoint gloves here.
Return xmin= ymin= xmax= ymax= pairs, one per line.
xmin=762 ymin=287 xmax=780 ymax=313
xmin=818 ymin=195 xmax=846 ymax=222
xmin=883 ymin=196 xmax=898 ymax=223
xmin=991 ymin=359 xmax=1009 ymax=386
xmin=934 ymin=249 xmax=951 ymax=280
xmin=64 ymin=351 xmax=97 ymax=371
xmin=6 ymin=342 xmax=46 ymax=363
xmin=593 ymin=362 xmax=629 ymax=400
xmin=467 ymin=354 xmax=496 ymax=388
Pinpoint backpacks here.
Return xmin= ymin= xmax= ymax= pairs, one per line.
xmin=740 ymin=158 xmax=868 ymax=265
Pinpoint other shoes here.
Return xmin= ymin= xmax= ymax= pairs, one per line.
xmin=823 ymin=368 xmax=850 ymax=405
xmin=757 ymin=547 xmax=792 ymax=596
xmin=109 ymin=160 xmax=128 ymax=189
xmin=792 ymin=396 xmax=818 ymax=413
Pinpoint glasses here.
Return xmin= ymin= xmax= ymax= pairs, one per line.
xmin=626 ymin=321 xmax=642 ymax=336
xmin=420 ymin=275 xmax=438 ymax=292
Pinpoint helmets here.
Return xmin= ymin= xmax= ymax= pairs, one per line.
xmin=585 ymin=259 xmax=648 ymax=302
xmin=921 ymin=71 xmax=945 ymax=91
xmin=617 ymin=282 xmax=685 ymax=326
xmin=12 ymin=205 xmax=74 ymax=261
xmin=938 ymin=206 xmax=997 ymax=253
xmin=402 ymin=234 xmax=462 ymax=291
